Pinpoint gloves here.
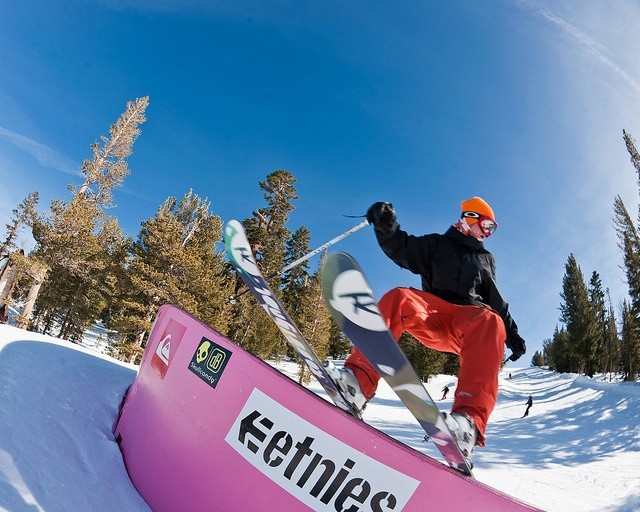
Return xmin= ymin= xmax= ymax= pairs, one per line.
xmin=366 ymin=201 xmax=397 ymax=228
xmin=506 ymin=331 xmax=526 ymax=362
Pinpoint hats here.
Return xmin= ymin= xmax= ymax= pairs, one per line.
xmin=461 ymin=196 xmax=494 ymax=226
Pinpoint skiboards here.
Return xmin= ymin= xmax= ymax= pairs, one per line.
xmin=224 ymin=219 xmax=472 ymax=476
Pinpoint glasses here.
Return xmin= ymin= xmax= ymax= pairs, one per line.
xmin=461 ymin=211 xmax=498 ymax=238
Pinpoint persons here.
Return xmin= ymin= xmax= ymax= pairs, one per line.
xmin=440 ymin=385 xmax=449 ymax=401
xmin=520 ymin=395 xmax=533 ymax=419
xmin=321 ymin=194 xmax=527 ymax=468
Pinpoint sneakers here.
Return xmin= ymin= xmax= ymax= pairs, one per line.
xmin=442 ymin=410 xmax=479 ymax=457
xmin=322 ymin=359 xmax=369 ymax=413
